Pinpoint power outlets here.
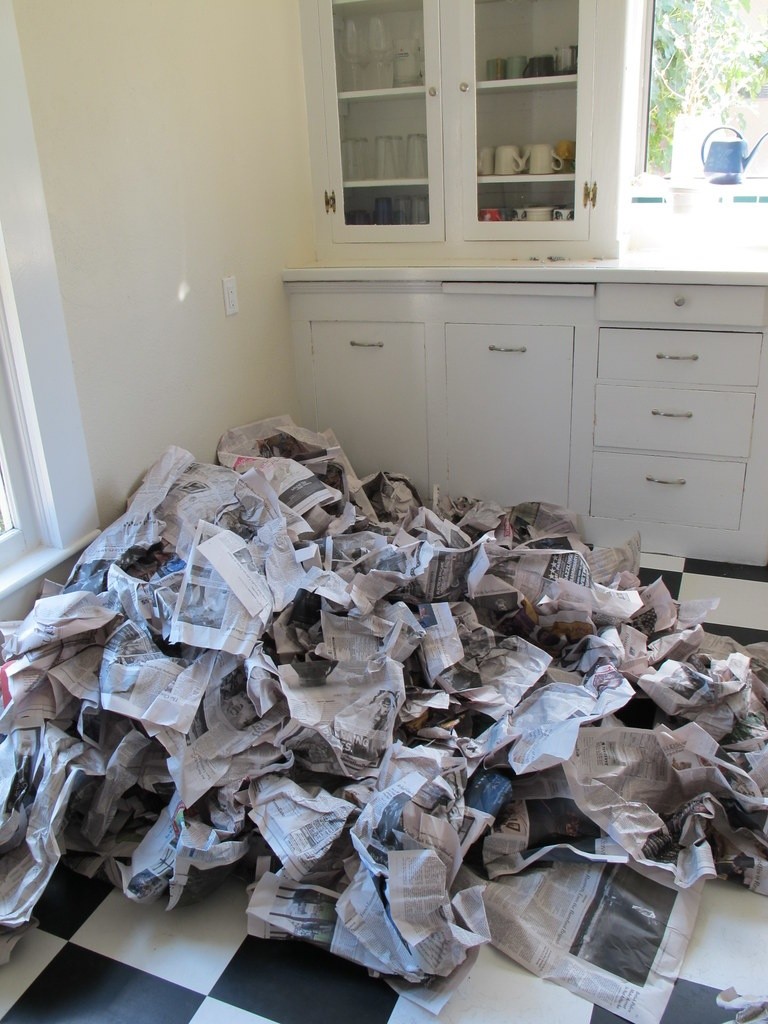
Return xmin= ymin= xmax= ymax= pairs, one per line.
xmin=220 ymin=275 xmax=241 ymax=317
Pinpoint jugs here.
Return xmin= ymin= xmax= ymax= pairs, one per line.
xmin=701 ymin=127 xmax=768 ymax=185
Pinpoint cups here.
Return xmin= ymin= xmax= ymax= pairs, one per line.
xmin=523 ymin=56 xmax=555 ymax=78
xmin=348 ymin=210 xmax=372 ymax=225
xmin=411 ymin=194 xmax=429 ymax=225
xmin=486 ymin=59 xmax=507 ymax=81
xmin=520 ymin=144 xmax=535 ymax=175
xmin=557 ymin=160 xmax=576 ymax=174
xmin=394 ymin=195 xmax=411 ymax=224
xmin=557 ymin=140 xmax=575 ymax=160
xmin=555 ymin=44 xmax=577 ymax=75
xmin=479 ymin=208 xmax=501 ymax=221
xmin=403 ymin=135 xmax=425 ymax=179
xmin=511 ymin=208 xmax=526 ymax=221
xmin=528 ymin=144 xmax=564 ymax=175
xmin=373 ymin=197 xmax=393 ymax=225
xmin=495 ymin=145 xmax=523 ymax=176
xmin=371 ymin=137 xmax=396 ymax=179
xmin=506 ymin=56 xmax=528 ymax=79
xmin=553 ymin=209 xmax=574 ymax=221
xmin=354 ymin=138 xmax=371 ymax=179
xmin=391 ymin=135 xmax=406 ymax=178
xmin=341 ymin=138 xmax=359 ymax=181
xmin=477 ymin=146 xmax=495 ymax=174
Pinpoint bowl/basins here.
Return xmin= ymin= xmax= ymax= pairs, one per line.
xmin=524 ymin=206 xmax=554 ymax=221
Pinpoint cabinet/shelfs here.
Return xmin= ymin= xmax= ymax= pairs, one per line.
xmin=268 ymin=9 xmax=768 ymax=570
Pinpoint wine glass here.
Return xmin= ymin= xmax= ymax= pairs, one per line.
xmin=340 ymin=18 xmax=365 ymax=91
xmin=367 ymin=14 xmax=397 ymax=90
